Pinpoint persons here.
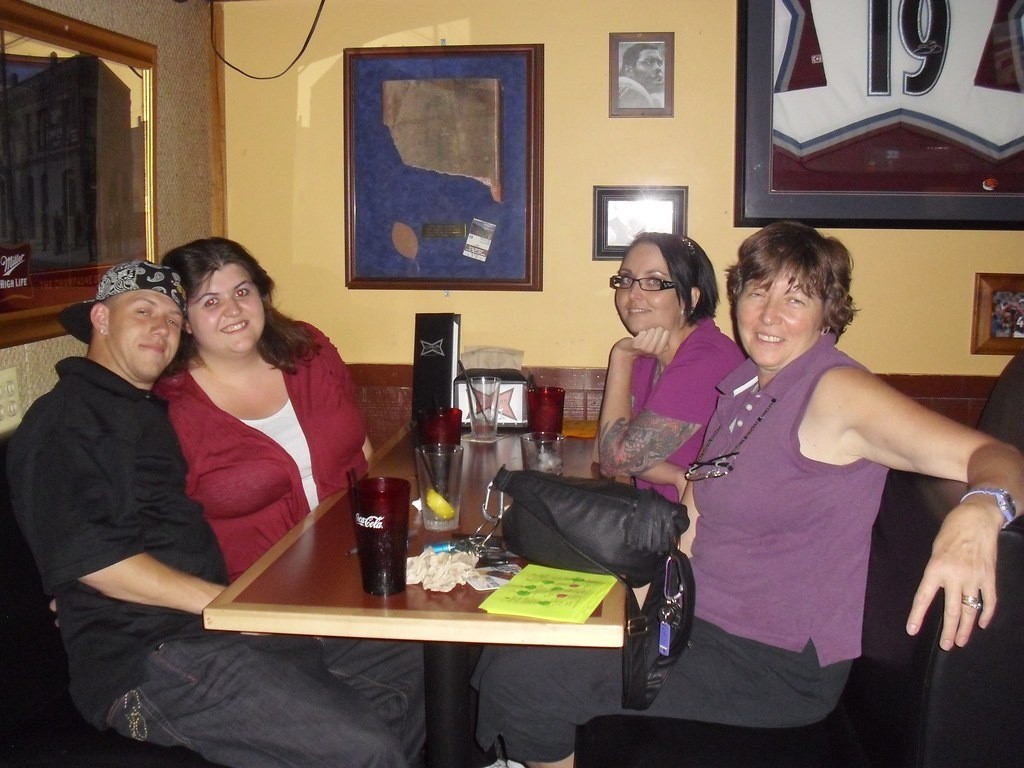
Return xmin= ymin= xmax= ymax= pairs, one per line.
xmin=50 ymin=238 xmax=374 ymax=627
xmin=5 ymin=259 xmax=427 ymax=768
xmin=591 ymin=232 xmax=748 ymax=504
xmin=618 ymin=43 xmax=665 ymax=109
xmin=470 ymin=220 xmax=1024 ymax=768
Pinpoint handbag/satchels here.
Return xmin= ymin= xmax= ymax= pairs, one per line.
xmin=478 ymin=464 xmax=698 ymax=712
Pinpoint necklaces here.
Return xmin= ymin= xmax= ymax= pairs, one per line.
xmin=685 ymin=399 xmax=776 ymax=481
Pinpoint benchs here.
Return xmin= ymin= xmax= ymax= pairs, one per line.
xmin=0 ymin=427 xmax=222 ymax=768
xmin=574 ymin=467 xmax=1024 ymax=768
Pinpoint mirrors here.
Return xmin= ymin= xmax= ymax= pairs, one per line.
xmin=0 ymin=0 xmax=158 ymax=354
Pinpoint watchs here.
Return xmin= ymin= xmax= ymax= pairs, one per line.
xmin=960 ymin=487 xmax=1016 ymax=529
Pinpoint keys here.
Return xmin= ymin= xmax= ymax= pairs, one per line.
xmin=658 ymin=603 xmax=695 ymax=651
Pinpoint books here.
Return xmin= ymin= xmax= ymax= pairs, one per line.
xmin=412 ymin=313 xmax=461 ymax=423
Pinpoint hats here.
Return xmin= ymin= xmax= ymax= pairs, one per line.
xmin=58 ymin=258 xmax=186 ymax=347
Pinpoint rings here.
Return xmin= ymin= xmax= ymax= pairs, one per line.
xmin=961 ymin=595 xmax=983 ymax=610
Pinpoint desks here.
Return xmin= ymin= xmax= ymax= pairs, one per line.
xmin=203 ymin=422 xmax=628 ymax=768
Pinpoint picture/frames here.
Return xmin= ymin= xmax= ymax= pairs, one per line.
xmin=970 ymin=273 xmax=1024 ymax=355
xmin=344 ymin=44 xmax=543 ymax=291
xmin=734 ymin=0 xmax=1024 ymax=229
xmin=609 ymin=31 xmax=674 ymax=118
xmin=593 ymin=186 xmax=688 ymax=261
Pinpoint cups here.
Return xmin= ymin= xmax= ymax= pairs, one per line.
xmin=466 ymin=376 xmax=501 ymax=440
xmin=520 ymin=431 xmax=564 ymax=477
xmin=414 ymin=444 xmax=464 ymax=530
xmin=419 ymin=408 xmax=462 ymax=495
xmin=349 ymin=478 xmax=411 ymax=594
xmin=529 ymin=386 xmax=564 ymax=444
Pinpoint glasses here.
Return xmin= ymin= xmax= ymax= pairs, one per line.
xmin=685 ymin=451 xmax=741 ymax=480
xmin=609 ymin=273 xmax=675 ymax=291
